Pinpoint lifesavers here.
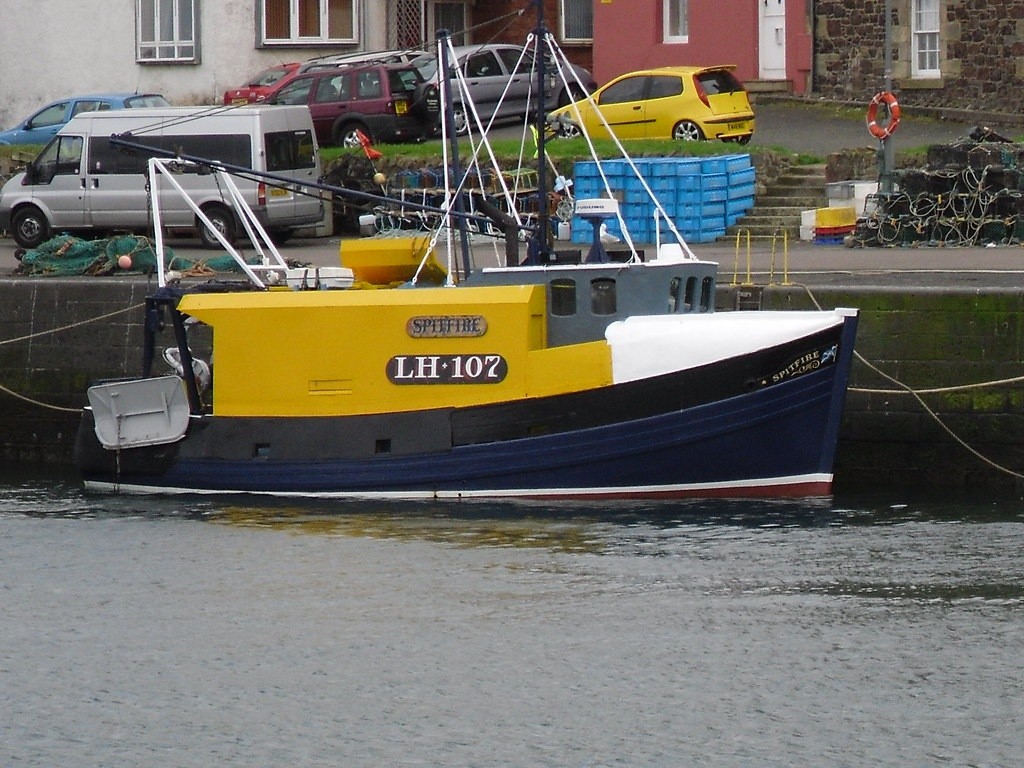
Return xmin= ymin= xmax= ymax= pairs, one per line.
xmin=867 ymin=92 xmax=900 ymax=139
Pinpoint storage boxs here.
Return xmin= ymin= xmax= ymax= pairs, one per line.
xmin=570 ymin=153 xmax=756 ymax=245
xmin=800 ymin=207 xmax=857 ymax=244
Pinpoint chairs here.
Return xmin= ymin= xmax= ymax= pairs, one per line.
xmin=319 ymin=85 xmax=338 ymax=103
xmin=361 ymin=80 xmax=379 ymax=95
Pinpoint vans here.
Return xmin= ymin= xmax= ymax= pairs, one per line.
xmin=0 ymin=103 xmax=325 ymax=251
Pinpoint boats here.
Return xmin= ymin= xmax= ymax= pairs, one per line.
xmin=69 ymin=0 xmax=862 ymax=503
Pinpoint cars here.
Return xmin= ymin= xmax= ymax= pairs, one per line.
xmin=223 ymin=49 xmax=430 ymax=106
xmin=0 ymin=91 xmax=176 ymax=147
xmin=545 ymin=64 xmax=756 ymax=147
xmin=400 ymin=43 xmax=593 ymax=138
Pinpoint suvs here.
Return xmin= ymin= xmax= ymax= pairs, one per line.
xmin=256 ymin=60 xmax=440 ymax=151
